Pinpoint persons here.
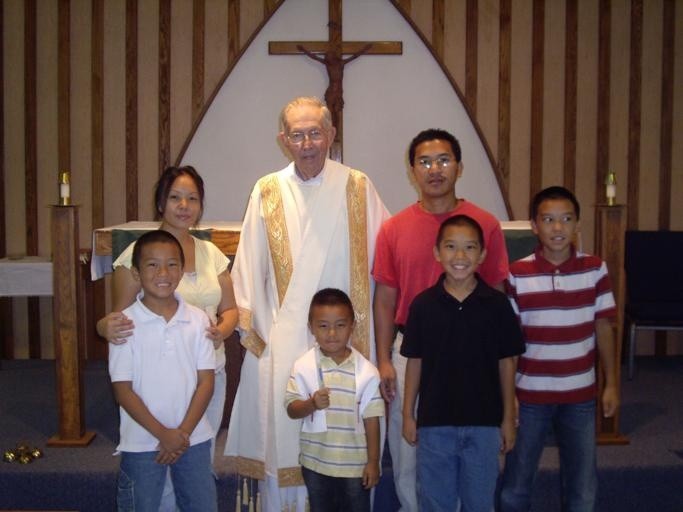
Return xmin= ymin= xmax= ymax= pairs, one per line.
xmin=226 ymin=95 xmax=403 ymax=511
xmin=400 ymin=213 xmax=526 ymax=511
xmin=370 ymin=125 xmax=512 ymax=512
xmin=95 ymin=163 xmax=237 ymax=443
xmin=283 ymin=286 xmax=385 ymax=511
xmin=490 ymin=184 xmax=621 ymax=512
xmin=105 ymin=228 xmax=222 ymax=512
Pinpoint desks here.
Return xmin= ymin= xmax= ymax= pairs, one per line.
xmin=0 ymin=252 xmax=92 ymax=365
xmin=90 ymin=219 xmax=243 ymax=257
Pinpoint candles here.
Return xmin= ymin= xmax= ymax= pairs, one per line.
xmin=604 ymin=173 xmax=617 ymax=207
xmin=59 ymin=171 xmax=71 ymax=207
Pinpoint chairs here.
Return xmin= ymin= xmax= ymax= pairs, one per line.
xmin=615 ymin=230 xmax=682 ymax=383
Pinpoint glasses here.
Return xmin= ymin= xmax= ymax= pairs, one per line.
xmin=281 ymin=131 xmax=328 ymax=142
xmin=412 ymin=157 xmax=458 ymax=168
